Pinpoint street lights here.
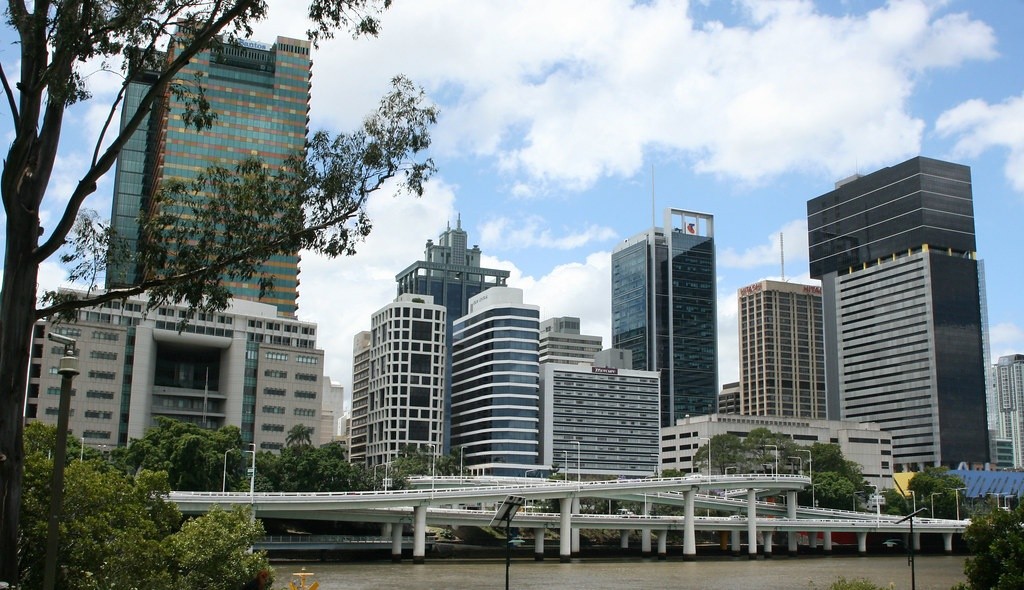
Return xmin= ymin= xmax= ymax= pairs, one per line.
xmin=945 ymin=487 xmax=969 ymax=521
xmin=795 ymin=449 xmax=812 ymax=484
xmin=763 ymin=445 xmax=778 ymax=482
xmin=459 ymin=446 xmax=468 ymax=485
xmin=699 ymin=438 xmax=711 ymax=484
xmin=787 ymin=457 xmax=802 ymax=475
xmin=724 ymin=467 xmax=737 ymax=500
xmin=245 ymin=450 xmax=255 ymax=494
xmin=571 ymin=442 xmax=580 ymax=490
xmin=813 ymin=484 xmax=820 ymax=513
xmin=853 ymin=491 xmax=864 ymax=511
xmin=524 ymin=469 xmax=534 ymax=516
xmin=427 ymin=444 xmax=436 ymax=499
xmin=562 ymin=451 xmax=568 ymax=486
xmin=223 ymin=449 xmax=231 ymax=496
xmin=863 ymin=485 xmax=889 ymax=528
xmin=384 ymin=463 xmax=388 ymax=494
xmin=908 ymin=490 xmax=916 ymax=520
xmin=931 ymin=493 xmax=942 ymax=519
